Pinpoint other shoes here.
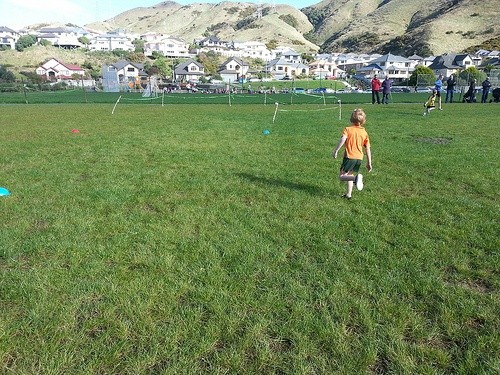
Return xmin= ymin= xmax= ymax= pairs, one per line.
xmin=438 ymin=108 xmax=443 ymax=110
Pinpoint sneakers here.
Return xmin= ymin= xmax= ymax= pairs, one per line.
xmin=341 ymin=194 xmax=351 ymax=200
xmin=353 ymin=174 xmax=363 ymax=191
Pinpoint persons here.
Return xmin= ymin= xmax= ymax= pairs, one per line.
xmin=381 ymin=76 xmax=392 ymax=104
xmin=445 ymin=75 xmax=456 ymax=104
xmin=422 ymin=90 xmax=437 ymax=116
xmin=372 ymin=74 xmax=381 ymax=104
xmin=469 ymin=79 xmax=476 ymax=103
xmin=333 ymin=108 xmax=372 ymax=199
xmin=424 ymin=75 xmax=443 ymax=110
xmin=162 ymin=82 xmax=276 ymax=94
xmin=481 ymin=77 xmax=492 ymax=104
